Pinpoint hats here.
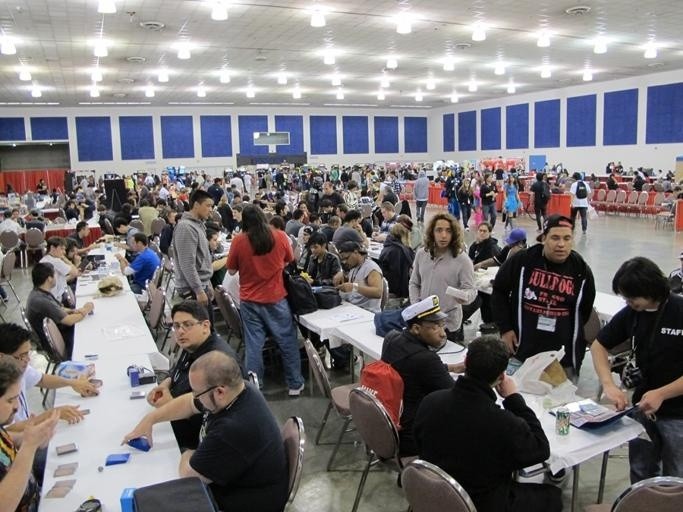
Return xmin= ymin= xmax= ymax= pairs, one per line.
xmin=400 ymin=295 xmax=448 ymax=322
xmin=339 ymin=240 xmax=368 ymax=254
xmin=536 ymin=214 xmax=575 ymax=242
xmin=505 ymin=229 xmax=526 ymax=245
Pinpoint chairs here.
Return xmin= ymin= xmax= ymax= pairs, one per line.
xmin=414 ymin=173 xmax=682 ymax=222
xmin=608 ymin=473 xmax=682 ymax=512
xmin=0 ymin=219 xmax=658 ymax=512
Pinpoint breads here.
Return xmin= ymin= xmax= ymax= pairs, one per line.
xmin=540 ymin=358 xmax=568 ymax=389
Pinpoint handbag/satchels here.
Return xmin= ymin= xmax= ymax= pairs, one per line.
xmin=374 ymin=312 xmax=408 ymax=337
xmin=540 ymin=182 xmax=549 ymax=201
xmin=283 ymin=270 xmax=342 ymax=315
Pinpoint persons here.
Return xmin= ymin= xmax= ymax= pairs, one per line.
xmin=0 ymin=354 xmax=62 ymax=511
xmin=147 ymin=300 xmax=248 ymax=459
xmin=119 ymin=350 xmax=287 ymax=511
xmin=380 ymin=296 xmax=467 ymax=455
xmin=407 ymin=213 xmax=479 ymax=340
xmin=94 ymin=161 xmax=417 ymax=395
xmin=412 ymin=335 xmax=563 ymax=512
xmin=466 ymin=219 xmax=530 ymax=334
xmin=489 ymin=215 xmax=596 ymax=479
xmin=0 ymin=321 xmax=99 ymax=434
xmin=22 ymin=261 xmax=93 ymax=361
xmin=589 ymin=257 xmax=682 ymax=485
xmin=413 ymin=159 xmax=682 ymax=234
xmin=0 ymin=168 xmax=93 ymax=309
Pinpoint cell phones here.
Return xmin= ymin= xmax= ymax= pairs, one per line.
xmin=138 ymin=376 xmax=155 ymax=385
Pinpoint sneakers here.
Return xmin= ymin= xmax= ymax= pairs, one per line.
xmin=288 ymin=383 xmax=305 ymax=396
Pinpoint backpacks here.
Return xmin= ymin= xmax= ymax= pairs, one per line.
xmin=361 ymin=332 xmax=428 ymax=431
xmin=576 ymin=181 xmax=587 ymax=198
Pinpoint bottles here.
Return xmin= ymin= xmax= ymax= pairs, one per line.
xmin=461 ymin=319 xmax=476 ymax=340
xmin=477 ymin=322 xmax=500 ymax=339
xmin=105 ymin=241 xmax=112 ymax=257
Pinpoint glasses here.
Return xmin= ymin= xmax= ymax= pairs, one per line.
xmin=192 ymin=384 xmax=225 ymax=400
xmin=170 ymin=319 xmax=204 ymax=330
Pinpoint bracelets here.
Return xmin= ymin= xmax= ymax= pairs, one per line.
xmin=79 ymin=311 xmax=85 ymax=317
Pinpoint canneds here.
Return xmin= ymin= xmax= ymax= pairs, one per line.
xmin=555 ymin=407 xmax=571 ymax=437
